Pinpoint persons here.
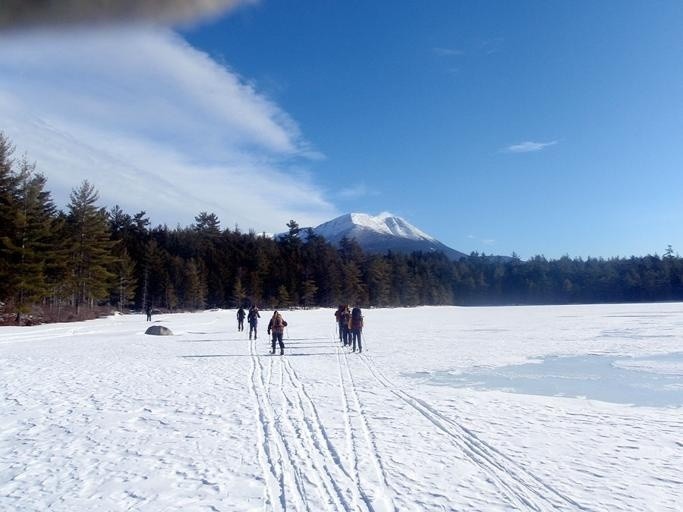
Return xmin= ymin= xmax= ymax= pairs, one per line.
xmin=335 ymin=303 xmax=364 ymax=353
xmin=247 ymin=304 xmax=261 ymax=339
xmin=146 ymin=306 xmax=152 ymax=321
xmin=268 ymin=310 xmax=287 ymax=355
xmin=236 ymin=306 xmax=246 ymax=332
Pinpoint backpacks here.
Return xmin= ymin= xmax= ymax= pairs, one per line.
xmin=352 ymin=308 xmax=360 ymax=329
xmin=272 ymin=314 xmax=284 ymax=328
